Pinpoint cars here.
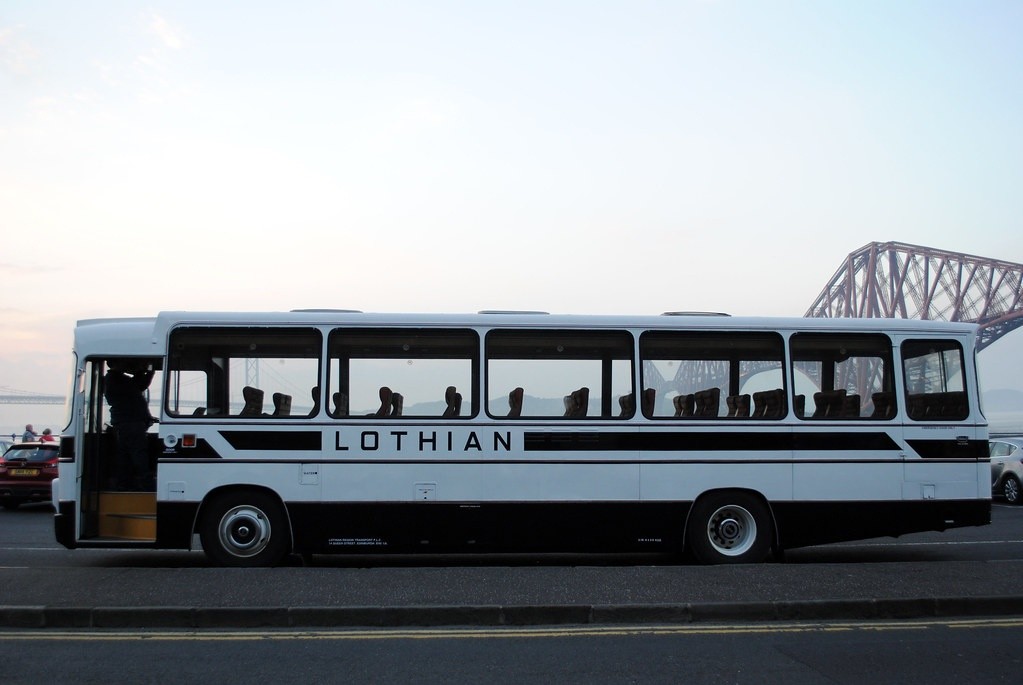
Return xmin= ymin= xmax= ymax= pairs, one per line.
xmin=0 ymin=440 xmax=61 ymax=509
xmin=988 ymin=436 xmax=1023 ymax=505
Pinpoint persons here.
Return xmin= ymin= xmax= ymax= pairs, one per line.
xmin=103 ymin=359 xmax=156 ymax=489
xmin=39 ymin=430 xmax=55 ymax=456
xmin=22 ymin=424 xmax=37 ymax=455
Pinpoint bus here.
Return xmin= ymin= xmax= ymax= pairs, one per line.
xmin=49 ymin=307 xmax=995 ymax=570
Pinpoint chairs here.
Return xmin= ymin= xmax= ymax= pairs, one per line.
xmin=191 ymin=386 xmax=967 ymax=421
